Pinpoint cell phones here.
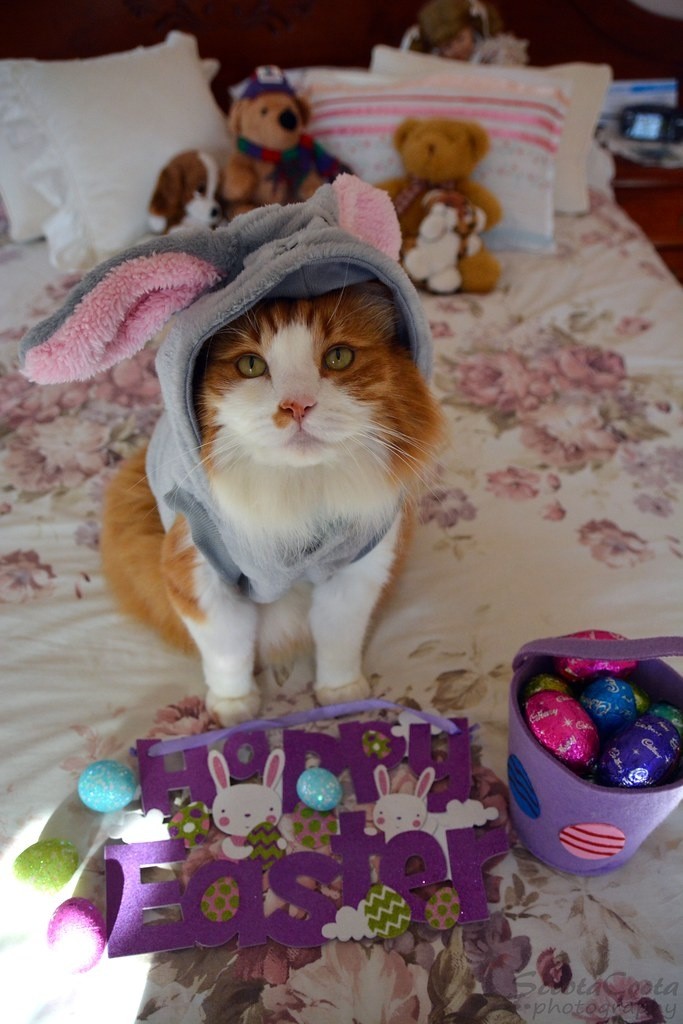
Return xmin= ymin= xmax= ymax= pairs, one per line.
xmin=622 ymin=102 xmax=683 ymax=144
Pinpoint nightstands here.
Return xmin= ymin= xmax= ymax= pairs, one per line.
xmin=610 ymin=153 xmax=682 ymax=288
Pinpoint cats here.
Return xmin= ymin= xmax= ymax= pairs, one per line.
xmin=100 ymin=282 xmax=445 ymax=728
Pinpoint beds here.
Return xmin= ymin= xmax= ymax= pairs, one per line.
xmin=1 ymin=184 xmax=682 ymax=1024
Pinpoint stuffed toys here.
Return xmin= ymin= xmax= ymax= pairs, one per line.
xmin=146 ymin=0 xmax=529 ymax=296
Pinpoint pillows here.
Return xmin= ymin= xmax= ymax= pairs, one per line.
xmin=231 ymin=65 xmax=369 ymax=102
xmin=0 ymin=30 xmax=227 ymax=274
xmin=370 ymin=44 xmax=613 ymax=214
xmin=307 ymin=80 xmax=572 ymax=255
